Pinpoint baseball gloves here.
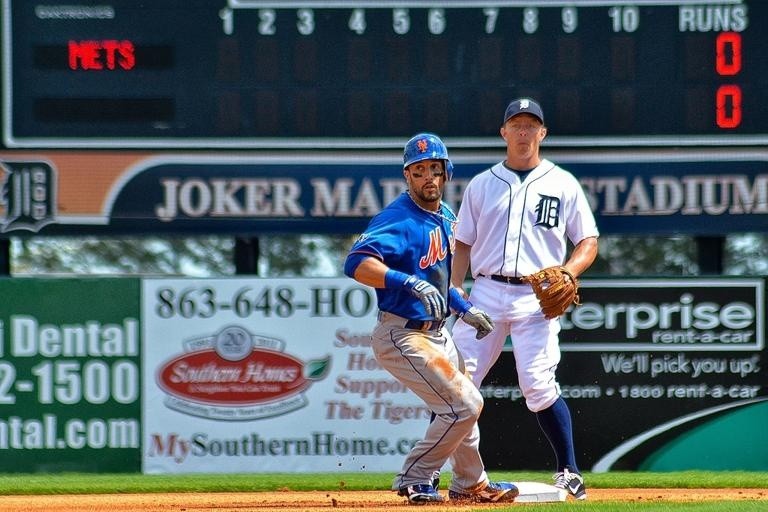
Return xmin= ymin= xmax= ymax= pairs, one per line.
xmin=522 ymin=266 xmax=579 ymax=320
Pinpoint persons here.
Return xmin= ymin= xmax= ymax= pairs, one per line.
xmin=344 ymin=134 xmax=519 ymax=504
xmin=431 ymin=97 xmax=599 ymax=501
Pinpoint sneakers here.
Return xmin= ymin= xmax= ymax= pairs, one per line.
xmin=397 ymin=484 xmax=444 ymax=505
xmin=449 ymin=482 xmax=519 ymax=503
xmin=552 ymin=468 xmax=586 ymax=501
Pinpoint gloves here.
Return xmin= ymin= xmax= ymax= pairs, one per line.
xmin=461 ymin=306 xmax=496 ymax=340
xmin=403 ymin=275 xmax=447 ymax=321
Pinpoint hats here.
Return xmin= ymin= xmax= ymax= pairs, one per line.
xmin=504 ymin=97 xmax=544 ymax=124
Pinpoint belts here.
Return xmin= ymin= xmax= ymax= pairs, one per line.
xmin=377 ymin=312 xmax=445 ymax=336
xmin=477 ymin=272 xmax=526 ymax=288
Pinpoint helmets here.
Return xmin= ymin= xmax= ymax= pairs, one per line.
xmin=403 ymin=134 xmax=449 ymax=170
